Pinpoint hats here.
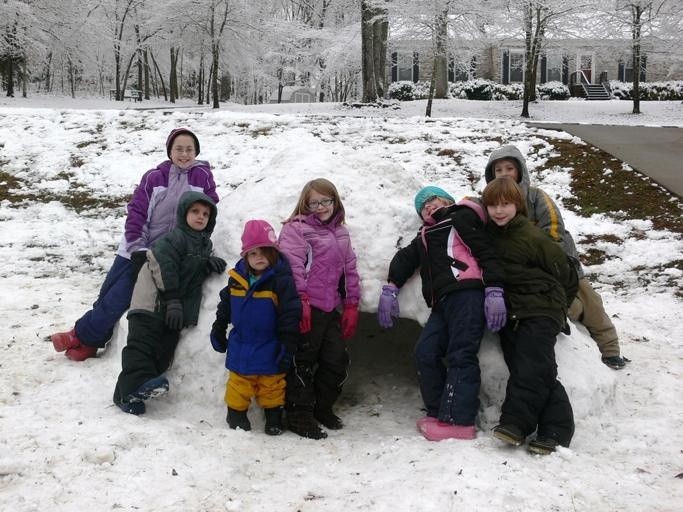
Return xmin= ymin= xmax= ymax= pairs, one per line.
xmin=165 ymin=128 xmax=201 ymax=158
xmin=415 ymin=186 xmax=454 ymax=214
xmin=240 ymin=219 xmax=280 ymax=259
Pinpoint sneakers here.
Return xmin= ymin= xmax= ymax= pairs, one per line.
xmin=227 ymin=407 xmax=250 ymax=430
xmin=65 ymin=344 xmax=98 ymax=361
xmin=493 ymin=422 xmax=526 ymax=446
xmin=51 ymin=329 xmax=81 ymax=352
xmin=602 ymin=354 xmax=626 ymax=370
xmin=286 ymin=407 xmax=328 ymax=440
xmin=416 ymin=415 xmax=475 ymax=440
xmin=530 ymin=434 xmax=561 ymax=456
xmin=136 ymin=377 xmax=171 ymax=399
xmin=316 ymin=401 xmax=344 ymax=429
xmin=264 ymin=406 xmax=283 ymax=435
xmin=114 ymin=392 xmax=145 ymax=415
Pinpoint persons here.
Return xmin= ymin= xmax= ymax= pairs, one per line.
xmin=113 ymin=191 xmax=228 ymax=416
xmin=51 ymin=128 xmax=219 ymax=362
xmin=277 ymin=178 xmax=360 ymax=440
xmin=209 ymin=219 xmax=302 ymax=436
xmin=481 ymin=176 xmax=579 ymax=458
xmin=377 ymin=186 xmax=506 ymax=442
xmin=485 ymin=145 xmax=626 ymax=369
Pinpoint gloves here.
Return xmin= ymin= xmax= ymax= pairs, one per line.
xmin=210 ymin=321 xmax=228 ymax=354
xmin=378 ymin=284 xmax=399 ymax=330
xmin=163 ymin=300 xmax=183 ymax=332
xmin=299 ymin=295 xmax=312 ymax=334
xmin=130 ymin=252 xmax=148 ymax=281
xmin=273 ymin=344 xmax=296 ymax=372
xmin=204 ymin=256 xmax=226 ymax=274
xmin=484 ymin=286 xmax=506 ymax=335
xmin=341 ymin=305 xmax=360 ymax=337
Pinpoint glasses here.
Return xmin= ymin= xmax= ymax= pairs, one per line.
xmin=309 ymin=200 xmax=334 ymax=210
